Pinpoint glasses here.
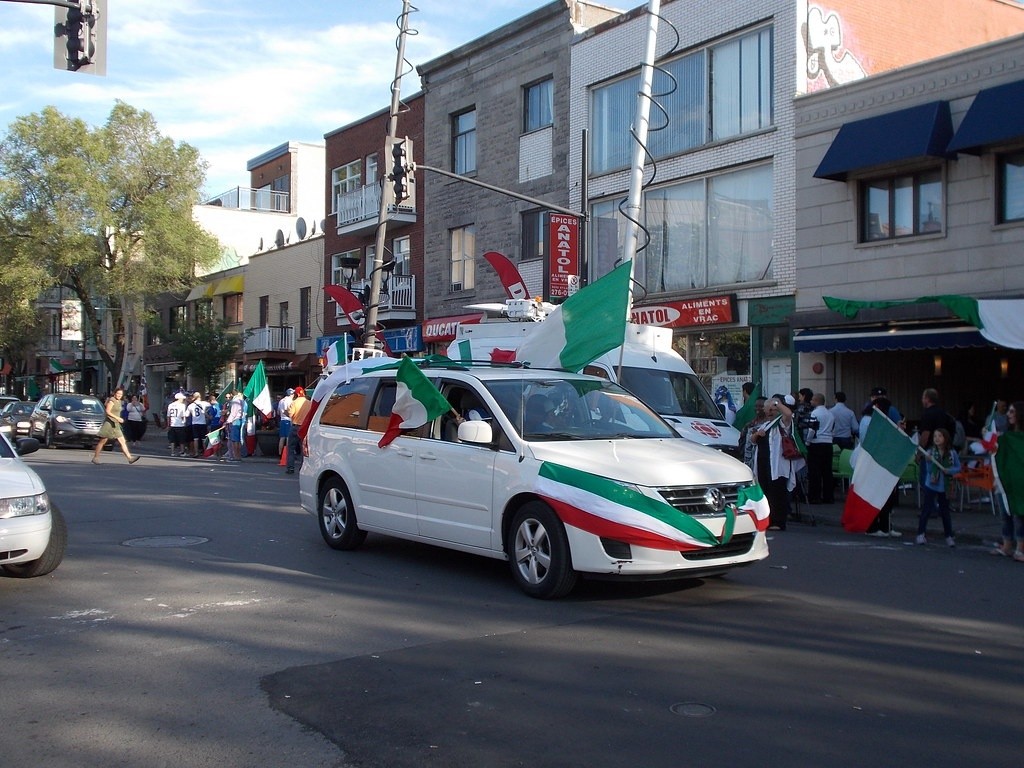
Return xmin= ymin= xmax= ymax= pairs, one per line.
xmin=225 ymin=397 xmax=231 ymax=398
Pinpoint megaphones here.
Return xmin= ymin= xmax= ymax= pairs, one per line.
xmin=353 ymin=311 xmax=363 ymax=320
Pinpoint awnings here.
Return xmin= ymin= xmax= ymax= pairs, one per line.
xmin=811 ymin=78 xmax=1024 ymax=183
xmin=184 ymin=274 xmax=244 ymax=302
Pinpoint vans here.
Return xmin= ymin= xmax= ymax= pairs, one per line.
xmin=295 ymin=363 xmax=770 ymax=600
xmin=442 ymin=297 xmax=742 ymax=461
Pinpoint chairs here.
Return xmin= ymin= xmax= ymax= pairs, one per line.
xmin=832 ymin=438 xmax=996 ymax=515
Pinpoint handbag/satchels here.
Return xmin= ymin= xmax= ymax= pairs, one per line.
xmin=777 ymin=424 xmax=803 ymax=460
xmin=141 ymin=416 xmax=149 ymax=424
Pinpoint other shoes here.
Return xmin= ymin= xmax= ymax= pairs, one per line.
xmin=945 ymin=537 xmax=956 ymax=548
xmin=915 ymin=533 xmax=927 ymax=544
xmin=889 ymin=530 xmax=902 ymax=538
xmin=91 ymin=458 xmax=101 ymax=465
xmin=128 ymin=455 xmax=140 ymax=465
xmin=285 ymin=467 xmax=295 ymax=473
xmin=864 ymin=530 xmax=889 ymax=538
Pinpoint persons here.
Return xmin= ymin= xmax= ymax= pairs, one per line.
xmin=714 ymin=380 xmax=1024 ymax=562
xmin=91 ymin=388 xmax=145 ymax=465
xmin=525 ymin=392 xmax=617 ymax=434
xmin=273 ymin=386 xmax=312 ymax=474
xmin=454 ymin=408 xmax=493 ymax=444
xmin=166 ymin=390 xmax=248 ymax=462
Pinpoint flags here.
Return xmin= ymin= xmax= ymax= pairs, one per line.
xmin=296 ymin=353 xmax=466 ymax=447
xmin=243 ymin=398 xmax=256 ymax=457
xmin=970 ymin=410 xmax=997 ymax=455
xmin=49 ymin=357 xmax=63 ymax=373
xmin=483 ymin=252 xmax=531 ymax=300
xmin=822 ymin=295 xmax=1024 ymax=349
xmin=842 ymin=410 xmax=918 ymax=535
xmin=140 ymin=366 xmax=149 ymax=409
xmin=216 ymin=378 xmax=242 ymax=406
xmin=323 ymin=340 xmax=346 ymax=369
xmin=506 ymin=260 xmax=631 ymax=373
xmin=202 ymin=430 xmax=220 ymax=457
xmin=242 ymin=360 xmax=273 ymax=419
xmin=322 ymin=285 xmax=393 ymax=357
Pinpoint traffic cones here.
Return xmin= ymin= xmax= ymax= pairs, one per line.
xmin=277 ymin=446 xmax=288 ymax=466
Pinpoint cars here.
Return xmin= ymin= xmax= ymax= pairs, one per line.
xmin=28 ymin=393 xmax=116 ymax=451
xmin=0 ymin=431 xmax=68 ymax=579
xmin=0 ymin=397 xmax=22 ymax=415
xmin=0 ymin=401 xmax=39 ymax=441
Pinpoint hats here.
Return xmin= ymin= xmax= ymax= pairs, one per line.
xmin=295 ymin=386 xmax=306 ymax=397
xmin=783 ymin=395 xmax=796 ymax=406
xmin=869 ymin=386 xmax=888 ymax=396
xmin=286 ymin=388 xmax=295 ymax=395
xmin=175 ymin=393 xmax=187 ymax=399
xmin=192 ymin=392 xmax=202 ymax=401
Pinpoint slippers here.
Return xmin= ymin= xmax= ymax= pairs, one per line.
xmin=1011 ymin=551 xmax=1024 ymax=562
xmin=990 ymin=548 xmax=1013 ymax=557
